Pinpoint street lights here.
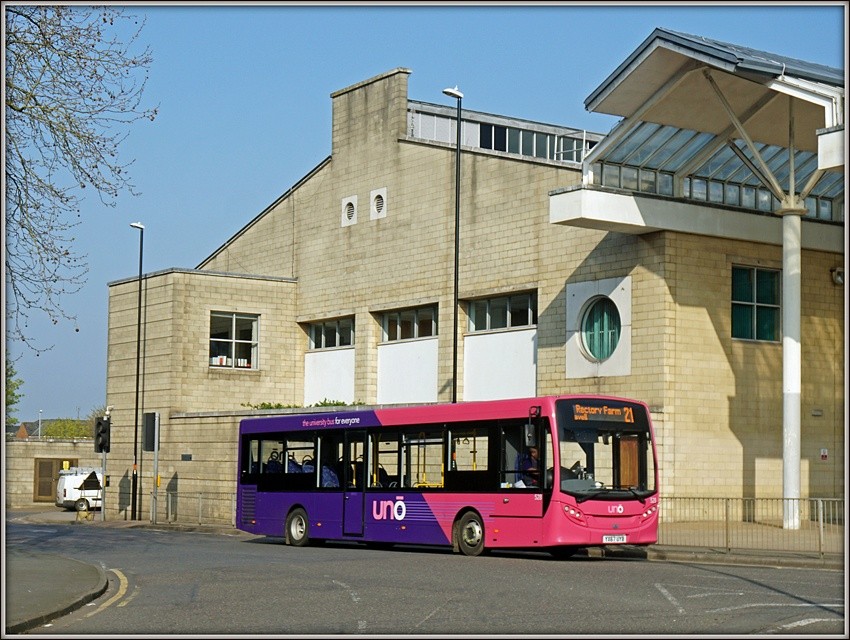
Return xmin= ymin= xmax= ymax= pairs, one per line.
xmin=38 ymin=409 xmax=44 ymax=440
xmin=442 ymin=86 xmax=463 ymax=403
xmin=129 ymin=222 xmax=145 ymax=521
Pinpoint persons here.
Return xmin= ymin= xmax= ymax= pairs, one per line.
xmin=378 ymin=468 xmax=389 ymax=488
xmin=307 ymin=446 xmax=335 ymax=473
xmin=521 ymin=447 xmax=539 ymax=485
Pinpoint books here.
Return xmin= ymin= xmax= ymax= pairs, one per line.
xmin=210 ymin=355 xmax=247 ymax=366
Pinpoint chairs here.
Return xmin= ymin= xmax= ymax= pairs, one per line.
xmin=254 ymin=451 xmax=388 ymax=487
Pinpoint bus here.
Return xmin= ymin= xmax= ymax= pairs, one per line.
xmin=235 ymin=393 xmax=660 ymax=559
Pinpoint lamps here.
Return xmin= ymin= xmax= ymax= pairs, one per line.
xmin=830 ymin=268 xmax=844 ymax=284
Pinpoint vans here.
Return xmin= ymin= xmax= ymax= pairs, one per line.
xmin=55 ymin=474 xmax=103 ymax=512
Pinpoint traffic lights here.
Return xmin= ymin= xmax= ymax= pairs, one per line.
xmin=94 ymin=417 xmax=110 ymax=453
